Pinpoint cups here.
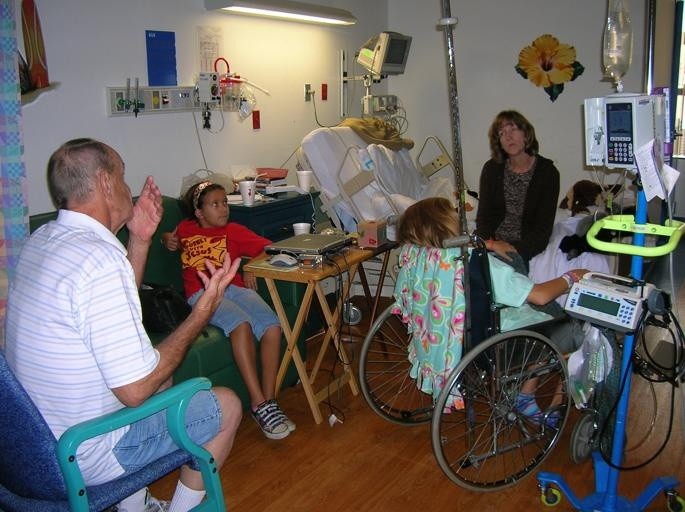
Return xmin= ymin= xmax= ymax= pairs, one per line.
xmin=293 ymin=222 xmax=312 ymax=235
xmin=295 ymin=169 xmax=313 ymax=194
xmin=239 ymin=180 xmax=258 ymax=207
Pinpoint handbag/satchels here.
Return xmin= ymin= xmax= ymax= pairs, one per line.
xmin=140 ymin=282 xmax=195 ymax=336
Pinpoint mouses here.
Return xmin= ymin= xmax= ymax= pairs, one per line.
xmin=269 ymin=253 xmax=299 ymax=267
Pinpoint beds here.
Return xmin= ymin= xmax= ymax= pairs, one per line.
xmin=297 ymin=126 xmax=618 ymax=325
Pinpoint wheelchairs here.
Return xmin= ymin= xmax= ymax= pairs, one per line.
xmin=356 ymin=231 xmax=597 ymax=491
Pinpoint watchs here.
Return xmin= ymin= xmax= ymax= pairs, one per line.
xmin=485 ymin=238 xmax=497 ymax=250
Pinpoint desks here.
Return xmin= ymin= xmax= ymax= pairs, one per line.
xmin=349 ymin=241 xmax=401 ymax=356
xmin=243 ymin=247 xmax=373 ymax=426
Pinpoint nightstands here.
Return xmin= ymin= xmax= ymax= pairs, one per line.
xmin=228 ymin=189 xmax=320 ymax=338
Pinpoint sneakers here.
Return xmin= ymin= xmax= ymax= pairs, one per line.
xmin=118 ymin=490 xmax=171 ymax=512
xmin=267 ymin=400 xmax=296 ymax=431
xmin=250 ymin=400 xmax=290 ymax=439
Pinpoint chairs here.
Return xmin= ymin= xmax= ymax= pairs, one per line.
xmin=0 ymin=352 xmax=227 ymax=512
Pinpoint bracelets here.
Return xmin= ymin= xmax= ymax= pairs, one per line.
xmin=561 ymin=269 xmax=581 ymax=288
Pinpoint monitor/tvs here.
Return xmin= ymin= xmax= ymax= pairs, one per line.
xmin=356 ymin=30 xmax=413 ymax=76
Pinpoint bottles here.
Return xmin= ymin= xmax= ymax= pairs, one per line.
xmin=222 ymin=79 xmax=242 ymax=112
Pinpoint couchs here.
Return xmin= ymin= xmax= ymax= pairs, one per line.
xmin=29 ymin=196 xmax=311 ymax=413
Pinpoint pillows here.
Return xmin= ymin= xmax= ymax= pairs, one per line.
xmin=367 ymin=142 xmax=423 ymax=197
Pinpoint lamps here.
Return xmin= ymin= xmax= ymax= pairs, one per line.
xmin=203 ymin=0 xmax=358 ymax=28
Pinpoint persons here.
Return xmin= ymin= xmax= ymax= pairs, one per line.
xmin=5 ymin=138 xmax=245 ymax=512
xmin=472 ymin=109 xmax=561 ymax=278
xmin=160 ymin=180 xmax=299 ymax=441
xmin=396 ymin=196 xmax=584 ymax=431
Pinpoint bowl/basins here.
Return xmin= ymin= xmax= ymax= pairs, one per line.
xmin=255 ymin=168 xmax=289 ymax=178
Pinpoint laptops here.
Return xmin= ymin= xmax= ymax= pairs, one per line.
xmin=263 ymin=233 xmax=353 ymax=258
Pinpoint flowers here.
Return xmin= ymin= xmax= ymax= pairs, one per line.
xmin=515 ymin=35 xmax=584 ymax=103
